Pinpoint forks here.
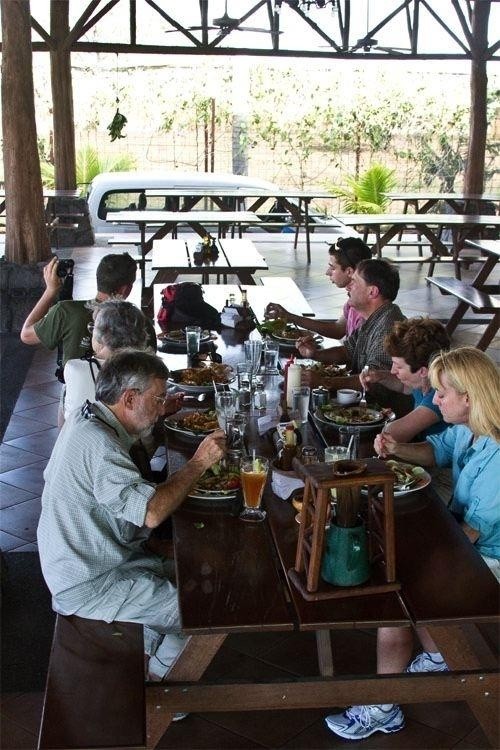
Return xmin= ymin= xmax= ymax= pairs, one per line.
xmin=358 ymin=365 xmax=369 ymax=416
xmin=182 ymin=394 xmax=211 ymax=401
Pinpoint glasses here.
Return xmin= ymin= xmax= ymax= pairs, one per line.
xmin=88 ymin=322 xmax=108 ymax=334
xmin=334 ymin=237 xmax=355 ymax=268
xmin=127 ymin=390 xmax=168 ymax=405
xmin=81 ymin=399 xmax=118 ymax=436
xmin=188 ymin=353 xmax=222 ymax=364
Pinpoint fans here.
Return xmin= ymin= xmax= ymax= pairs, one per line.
xmin=166 ymin=0 xmax=280 ymax=33
xmin=320 ymin=1 xmax=414 ymax=55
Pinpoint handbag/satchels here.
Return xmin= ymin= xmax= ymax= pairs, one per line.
xmin=158 ymin=282 xmax=221 ymax=332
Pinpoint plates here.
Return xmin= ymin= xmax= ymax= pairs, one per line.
xmin=314 ymin=402 xmax=395 ymax=431
xmin=272 ymin=325 xmax=318 ymax=340
xmin=186 ymin=462 xmax=238 ymax=498
xmin=158 ymin=329 xmax=217 ymax=345
xmin=360 ymin=461 xmax=431 ymax=497
xmin=164 ymin=408 xmax=220 ymax=436
xmin=167 ymin=368 xmax=236 ymax=392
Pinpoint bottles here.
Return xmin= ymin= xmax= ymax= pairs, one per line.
xmin=210 ymin=238 xmax=219 ymax=255
xmin=204 ymin=240 xmax=212 ymax=257
xmin=206 ymin=232 xmax=210 ymax=243
xmin=228 ymin=294 xmax=240 ymax=319
xmin=202 ymin=232 xmax=209 ymax=244
xmin=279 ymin=423 xmax=301 ymax=472
xmin=239 ymin=290 xmax=250 ymax=319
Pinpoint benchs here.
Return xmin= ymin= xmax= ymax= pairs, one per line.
xmin=108 ymin=237 xmax=151 ymax=245
xmin=425 ymin=276 xmax=499 ymax=310
xmin=252 ymin=223 xmax=341 ymax=228
xmin=406 ymin=224 xmax=496 ymax=229
xmin=0 ymin=222 xmax=78 ymax=228
xmin=261 ymin=277 xmax=314 ymax=315
xmin=37 ymin=612 xmax=147 ymax=749
xmin=145 ymin=223 xmax=250 ymax=239
xmin=51 ymin=211 xmax=85 ymax=217
xmin=324 ymin=240 xmax=455 ymax=256
xmin=256 ymin=213 xmax=325 ymax=216
xmin=372 ymin=257 xmax=489 ymax=281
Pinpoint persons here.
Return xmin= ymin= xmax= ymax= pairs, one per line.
xmin=22 ymin=254 xmax=157 ymax=430
xmin=359 ymin=317 xmax=450 ymax=442
xmin=36 ymin=352 xmax=227 ymax=721
xmin=325 ymin=348 xmax=500 ymax=739
xmin=295 ymin=259 xmax=406 ymax=388
xmin=264 ymin=237 xmax=372 ymax=339
xmin=63 ymin=301 xmax=159 ymax=463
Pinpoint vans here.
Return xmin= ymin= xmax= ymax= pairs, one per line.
xmin=86 ymin=171 xmax=361 ymax=235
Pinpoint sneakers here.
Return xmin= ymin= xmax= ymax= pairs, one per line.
xmin=404 ymin=652 xmax=450 ymax=672
xmin=323 ymin=704 xmax=406 ymax=742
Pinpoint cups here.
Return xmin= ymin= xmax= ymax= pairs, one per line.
xmin=244 ymin=340 xmax=262 ymax=375
xmin=238 ymin=457 xmax=270 ymax=521
xmin=293 ymin=387 xmax=309 ymax=422
xmin=186 ymin=326 xmax=200 ymax=356
xmin=265 ymin=341 xmax=279 ymax=374
xmin=215 ymin=392 xmax=238 ymax=433
xmin=337 ymin=389 xmax=362 ymax=403
xmin=237 ymin=363 xmax=253 ymax=388
xmin=338 ymin=426 xmax=359 ymax=459
xmin=325 ymin=446 xmax=351 ymax=503
